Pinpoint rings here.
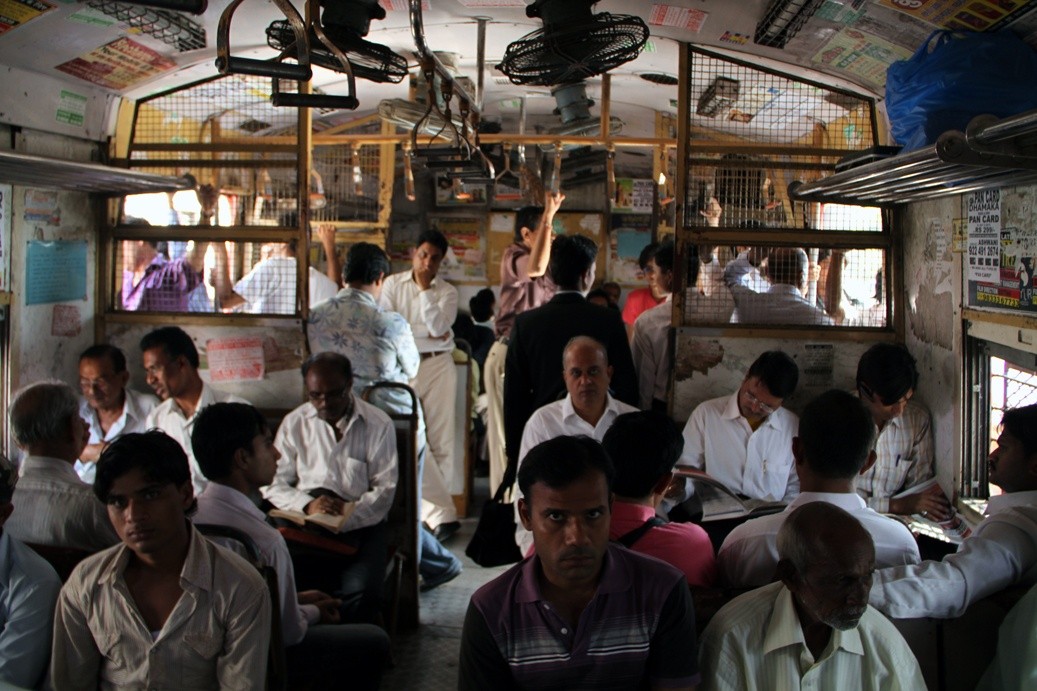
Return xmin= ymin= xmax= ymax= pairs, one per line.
xmin=921 ymin=510 xmax=928 ymax=516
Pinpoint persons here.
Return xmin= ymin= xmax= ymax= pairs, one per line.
xmin=526 ymin=410 xmax=715 ymax=589
xmin=867 ymin=402 xmax=1037 ymax=621
xmin=588 ymin=291 xmax=609 ymax=310
xmin=503 ymin=232 xmax=641 ymax=486
xmin=718 ymin=388 xmax=924 ymax=595
xmin=605 ymin=280 xmax=620 ymax=301
xmin=187 ymin=402 xmax=390 ymax=691
xmin=630 ymin=195 xmax=735 ymax=425
xmin=381 ymin=228 xmax=467 ymax=541
xmin=622 ymin=241 xmax=669 ymax=349
xmin=306 ymin=244 xmax=460 ymax=594
xmin=134 ymin=327 xmax=254 ymax=506
xmin=120 ymin=181 xmax=342 ymax=318
xmin=676 ymin=350 xmax=804 ymax=502
xmin=483 ymin=188 xmax=564 ymax=507
xmin=71 ymin=345 xmax=163 ymax=479
xmin=700 ymin=501 xmax=931 ymax=691
xmin=455 ymin=433 xmax=701 ymax=691
xmin=513 ymin=335 xmax=643 ymax=559
xmin=728 ymin=216 xmax=885 ymax=327
xmin=39 ymin=429 xmax=268 ymax=691
xmin=455 ymin=287 xmax=498 ymax=478
xmin=8 ymin=381 xmax=122 ymax=554
xmin=846 ymin=341 xmax=958 ymax=563
xmin=260 ymin=350 xmax=397 ymax=633
xmin=0 ymin=455 xmax=61 ymax=691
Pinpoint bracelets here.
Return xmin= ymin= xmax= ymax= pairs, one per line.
xmin=541 ymin=221 xmax=553 ymax=228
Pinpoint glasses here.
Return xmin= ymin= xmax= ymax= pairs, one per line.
xmin=743 ymin=388 xmax=775 ymax=415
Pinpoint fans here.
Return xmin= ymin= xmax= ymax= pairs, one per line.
xmin=491 ymin=0 xmax=652 ymax=83
xmin=265 ymin=0 xmax=410 ymax=79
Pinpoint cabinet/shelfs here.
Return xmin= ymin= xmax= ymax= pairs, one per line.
xmin=791 ymin=112 xmax=1037 ymax=205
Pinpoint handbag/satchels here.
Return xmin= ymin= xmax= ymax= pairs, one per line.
xmin=464 ymin=499 xmax=525 ymax=566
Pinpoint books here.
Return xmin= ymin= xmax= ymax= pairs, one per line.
xmin=270 ymin=500 xmax=355 ymax=534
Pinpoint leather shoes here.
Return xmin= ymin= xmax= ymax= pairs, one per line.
xmin=431 ymin=520 xmax=460 ymax=542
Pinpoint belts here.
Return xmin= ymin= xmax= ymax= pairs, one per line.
xmin=418 ymin=349 xmax=449 ymax=363
